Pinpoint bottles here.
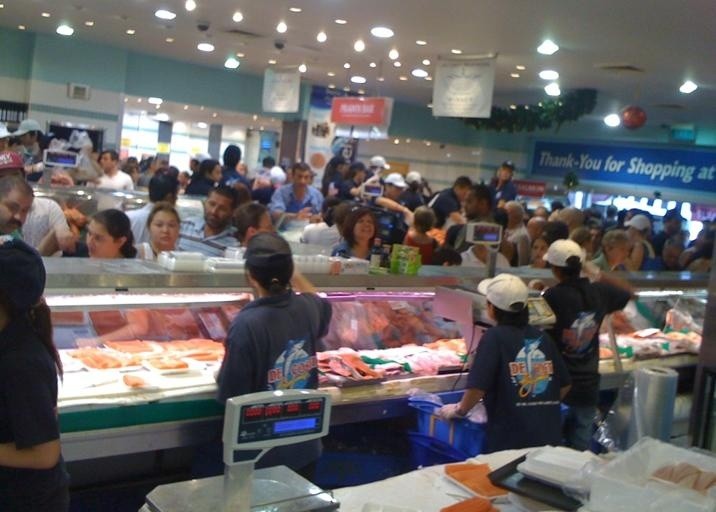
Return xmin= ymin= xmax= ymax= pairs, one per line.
xmin=370 ymin=238 xmax=385 ymax=268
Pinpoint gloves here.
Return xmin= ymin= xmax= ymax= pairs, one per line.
xmin=438 ymin=402 xmax=467 ymax=420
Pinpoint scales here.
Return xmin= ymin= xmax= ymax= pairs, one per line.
xmin=437 ymin=222 xmax=557 ymax=329
xmin=138 ymin=390 xmax=342 ymax=512
xmin=41 ymin=149 xmax=79 ymax=191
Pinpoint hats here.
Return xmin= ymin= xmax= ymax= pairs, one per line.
xmin=369 ymin=155 xmax=391 ymax=171
xmin=501 ymin=159 xmax=516 ymax=171
xmin=542 ymin=238 xmax=587 ymax=268
xmin=247 ymin=232 xmax=293 ymax=285
xmin=1 ymin=119 xmax=45 ymax=172
xmin=663 ymin=209 xmax=687 ymax=222
xmin=270 ymin=165 xmax=287 ymax=184
xmin=477 ymin=273 xmax=531 ymax=314
xmin=406 ymin=171 xmax=423 ymax=186
xmin=622 ymin=214 xmax=651 ymax=231
xmin=384 ymin=172 xmax=410 ymax=188
xmin=0 ymin=237 xmax=46 ymax=310
xmin=149 ymin=166 xmax=179 ymax=193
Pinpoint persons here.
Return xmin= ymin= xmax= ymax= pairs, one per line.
xmin=439 ymin=272 xmax=573 ymax=453
xmin=542 ymin=238 xmax=636 ymax=450
xmin=0 ymin=237 xmax=72 ymax=512
xmin=214 ymin=229 xmax=332 ymax=483
xmin=0 ymin=119 xmax=716 ymax=273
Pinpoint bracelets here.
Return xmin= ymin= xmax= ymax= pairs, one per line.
xmin=454 ymin=404 xmax=472 ymax=418
